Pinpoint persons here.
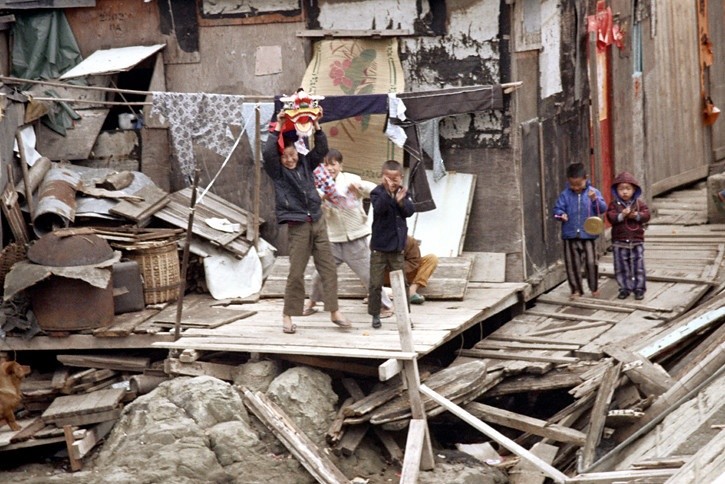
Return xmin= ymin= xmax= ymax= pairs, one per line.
xmin=303 ymin=148 xmax=438 ymax=304
xmin=367 ymin=160 xmax=414 ymax=328
xmin=607 ymin=172 xmax=650 ymax=300
xmin=262 ymin=110 xmax=352 ymax=333
xmin=552 ymin=163 xmax=607 ymax=300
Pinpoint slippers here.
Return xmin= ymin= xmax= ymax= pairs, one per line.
xmin=303 ymin=305 xmax=317 ymax=316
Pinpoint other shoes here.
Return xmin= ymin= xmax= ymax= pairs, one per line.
xmin=362 ymin=298 xmax=369 ymax=303
xmin=372 ymin=316 xmax=381 ymax=328
xmin=592 ymin=290 xmax=600 ymax=298
xmin=570 ymin=291 xmax=581 ymax=299
xmin=409 ymin=295 xmax=424 ymax=304
xmin=618 ymin=291 xmax=629 ymax=299
xmin=636 ymin=294 xmax=643 ymax=299
xmin=283 ymin=323 xmax=297 ymax=333
xmin=333 ymin=318 xmax=351 ymax=327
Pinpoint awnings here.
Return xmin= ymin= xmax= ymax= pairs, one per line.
xmin=59 ymin=44 xmax=166 ymax=80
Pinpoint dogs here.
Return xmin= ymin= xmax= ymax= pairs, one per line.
xmin=0 ymin=357 xmax=32 ymax=431
